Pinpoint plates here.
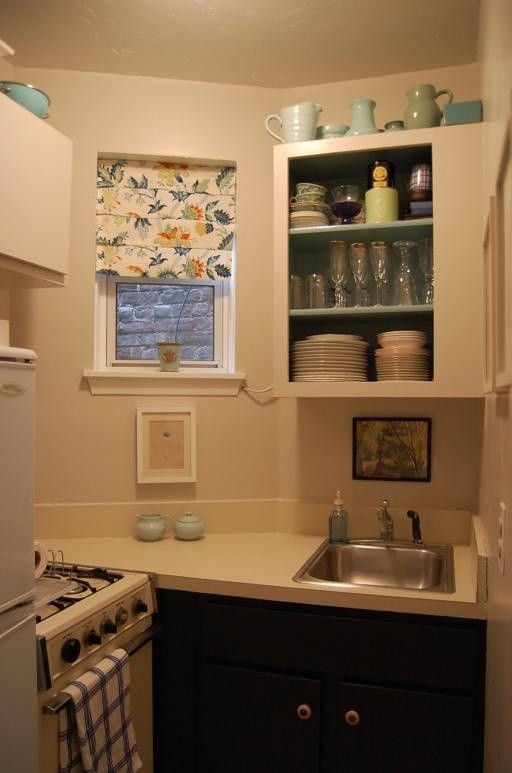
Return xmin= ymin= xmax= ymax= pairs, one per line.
xmin=289 ymin=202 xmax=333 ymax=228
xmin=291 ymin=329 xmax=435 ymax=381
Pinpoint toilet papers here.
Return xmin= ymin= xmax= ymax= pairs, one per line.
xmin=34 ymin=541 xmax=49 ymax=579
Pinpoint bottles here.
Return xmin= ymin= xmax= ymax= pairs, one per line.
xmin=344 ymin=98 xmax=404 ymax=137
xmin=366 ymin=159 xmax=399 ymax=223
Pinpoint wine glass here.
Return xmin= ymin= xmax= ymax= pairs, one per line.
xmin=329 ymin=239 xmax=432 ymax=307
xmin=329 ymin=185 xmax=363 ymax=224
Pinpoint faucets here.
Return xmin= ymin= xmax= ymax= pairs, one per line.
xmin=375 ymin=499 xmax=393 ymax=541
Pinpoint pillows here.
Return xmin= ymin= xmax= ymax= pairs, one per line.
xmin=58 ymin=648 xmax=144 ymax=773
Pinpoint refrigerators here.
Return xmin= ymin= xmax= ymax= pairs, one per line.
xmin=0 ymin=347 xmax=38 ymax=771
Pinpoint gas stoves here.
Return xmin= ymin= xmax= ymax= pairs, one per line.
xmin=36 ymin=548 xmax=155 ymax=692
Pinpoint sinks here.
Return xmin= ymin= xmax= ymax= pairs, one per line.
xmin=292 ymin=538 xmax=457 ymax=594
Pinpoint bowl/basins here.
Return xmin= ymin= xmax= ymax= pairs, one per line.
xmin=0 ymin=79 xmax=50 ymax=119
xmin=316 ymin=124 xmax=350 ymax=140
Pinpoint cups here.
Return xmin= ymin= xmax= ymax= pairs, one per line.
xmin=157 ymin=342 xmax=182 ymax=372
xmin=289 ymin=273 xmax=326 ymax=308
xmin=406 ymin=157 xmax=434 ymax=200
xmin=290 ymin=183 xmax=329 ymax=204
xmin=136 ymin=512 xmax=205 ymax=541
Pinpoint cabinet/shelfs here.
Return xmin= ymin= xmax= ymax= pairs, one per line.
xmin=273 ymin=123 xmax=488 ymax=398
xmin=0 ymin=91 xmax=74 ymax=290
xmin=200 ymin=601 xmax=486 ymax=773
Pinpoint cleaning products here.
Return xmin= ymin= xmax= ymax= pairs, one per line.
xmin=328 ymin=489 xmax=350 ymax=544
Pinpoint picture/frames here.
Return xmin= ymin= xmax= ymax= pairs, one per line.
xmin=136 ymin=406 xmax=196 ymax=484
xmin=352 ymin=417 xmax=432 ymax=481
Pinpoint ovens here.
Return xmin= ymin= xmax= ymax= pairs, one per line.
xmin=34 ymin=629 xmax=153 ymax=773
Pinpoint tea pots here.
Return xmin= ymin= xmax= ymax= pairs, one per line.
xmin=265 ymin=103 xmax=323 ymax=144
xmin=403 ymin=83 xmax=454 ymax=129
